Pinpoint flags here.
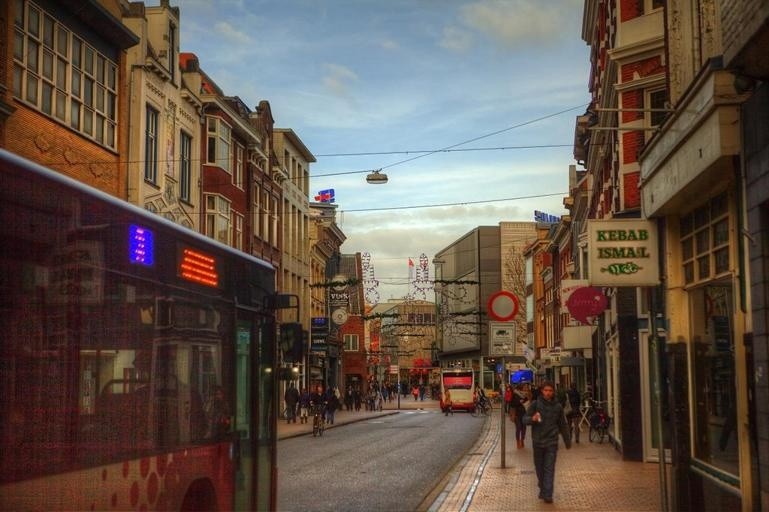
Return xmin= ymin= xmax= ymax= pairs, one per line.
xmin=409 ymin=258 xmax=414 ymax=267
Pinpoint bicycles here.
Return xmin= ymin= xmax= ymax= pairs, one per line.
xmin=470 ymin=395 xmax=493 ymax=418
xmin=308 ymin=401 xmax=327 ymax=436
xmin=588 ymin=396 xmax=609 ymax=445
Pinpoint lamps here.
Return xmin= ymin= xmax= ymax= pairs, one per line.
xmin=365 ymin=167 xmax=389 ymax=184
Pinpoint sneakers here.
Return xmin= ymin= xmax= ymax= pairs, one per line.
xmin=538 ymin=489 xmax=553 ymax=504
xmin=516 ymin=439 xmax=524 ymax=449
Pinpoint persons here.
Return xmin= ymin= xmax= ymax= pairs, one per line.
xmin=200 ymin=386 xmax=231 ymax=438
xmin=284 ymin=379 xmax=580 ymax=504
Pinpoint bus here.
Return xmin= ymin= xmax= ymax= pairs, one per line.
xmin=0 ymin=147 xmax=310 ymax=512
xmin=441 ymin=368 xmax=475 ymax=413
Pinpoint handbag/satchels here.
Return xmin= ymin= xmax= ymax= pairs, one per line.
xmin=509 ymin=407 xmax=517 ymax=423
xmin=563 ymin=399 xmax=573 ymax=416
